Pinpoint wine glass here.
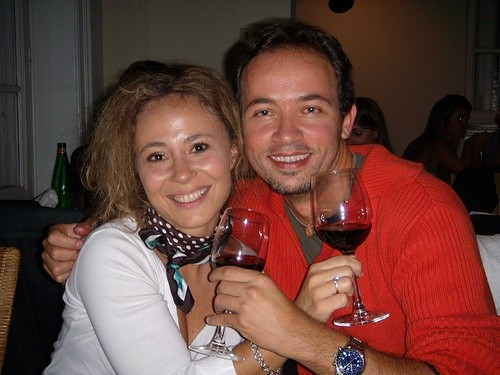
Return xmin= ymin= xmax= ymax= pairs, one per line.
xmin=187 ymin=206 xmax=271 ymax=363
xmin=310 ymin=168 xmax=389 ymax=327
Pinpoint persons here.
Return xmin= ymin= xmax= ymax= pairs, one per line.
xmin=403 ymin=95 xmax=500 ymax=184
xmin=345 ymin=97 xmax=391 ymax=153
xmin=42 ymin=17 xmax=500 ymax=374
xmin=42 ymin=65 xmax=364 ymax=375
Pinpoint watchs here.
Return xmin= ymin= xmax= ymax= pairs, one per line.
xmin=333 ymin=336 xmax=367 ymax=375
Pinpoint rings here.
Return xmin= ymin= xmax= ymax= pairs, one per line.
xmin=334 ymin=277 xmax=339 ymax=294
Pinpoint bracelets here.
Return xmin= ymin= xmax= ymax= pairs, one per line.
xmin=251 ymin=342 xmax=281 ymax=375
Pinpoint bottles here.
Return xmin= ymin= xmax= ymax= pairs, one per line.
xmin=50 ymin=143 xmax=74 ymax=210
